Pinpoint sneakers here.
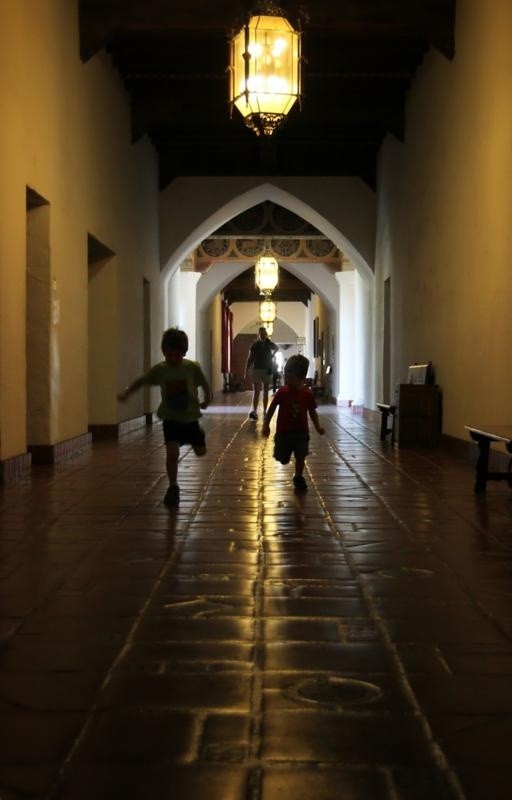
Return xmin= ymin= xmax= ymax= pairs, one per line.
xmin=293 ymin=476 xmax=308 ymax=490
xmin=249 ymin=412 xmax=258 ymax=420
xmin=164 ymin=485 xmax=179 ymax=505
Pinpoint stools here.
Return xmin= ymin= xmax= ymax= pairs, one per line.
xmin=466 ymin=423 xmax=512 ymax=493
xmin=376 ymin=401 xmax=398 ymax=447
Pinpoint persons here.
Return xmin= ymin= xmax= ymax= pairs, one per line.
xmin=243 ymin=327 xmax=278 ymax=420
xmin=115 ymin=328 xmax=212 ymax=508
xmin=261 ymin=353 xmax=326 ymax=490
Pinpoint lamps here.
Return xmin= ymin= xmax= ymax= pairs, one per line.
xmin=252 ymin=255 xmax=281 ymax=343
xmin=221 ymin=0 xmax=311 ymax=144
